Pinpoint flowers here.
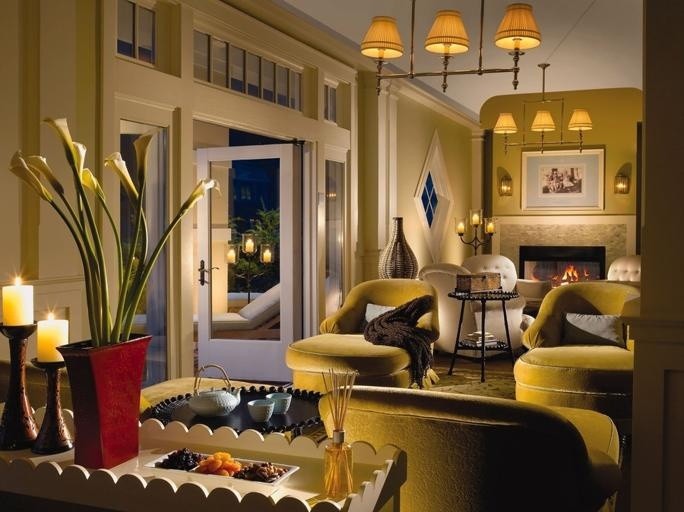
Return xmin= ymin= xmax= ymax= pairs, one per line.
xmin=8 ymin=115 xmax=225 ymax=347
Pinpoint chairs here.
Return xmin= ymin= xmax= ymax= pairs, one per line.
xmin=121 ymin=280 xmax=282 ymax=335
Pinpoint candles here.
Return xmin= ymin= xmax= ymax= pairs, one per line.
xmin=1 ymin=274 xmax=36 ymax=328
xmin=37 ymin=310 xmax=70 ymax=363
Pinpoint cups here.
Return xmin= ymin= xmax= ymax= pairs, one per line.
xmin=247 ymin=398 xmax=275 ymax=423
xmin=264 ymin=391 xmax=292 ymax=415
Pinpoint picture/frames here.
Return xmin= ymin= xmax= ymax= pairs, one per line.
xmin=520 ymin=146 xmax=606 ymax=212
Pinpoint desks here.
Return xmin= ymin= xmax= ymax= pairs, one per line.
xmin=140 ymin=376 xmax=330 ymax=446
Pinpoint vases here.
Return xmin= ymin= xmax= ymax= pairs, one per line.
xmin=54 ymin=332 xmax=155 ymax=471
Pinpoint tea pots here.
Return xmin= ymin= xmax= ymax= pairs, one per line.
xmin=186 ymin=364 xmax=242 ymax=418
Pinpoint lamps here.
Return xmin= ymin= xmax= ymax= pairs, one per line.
xmin=613 ymin=172 xmax=630 ymax=193
xmin=227 ymin=232 xmax=276 ymax=304
xmin=360 ymin=0 xmax=542 ymax=96
xmin=498 ymin=176 xmax=513 ymax=198
xmin=455 ymin=207 xmax=498 ymax=256
xmin=493 ymin=62 xmax=594 ymax=155
